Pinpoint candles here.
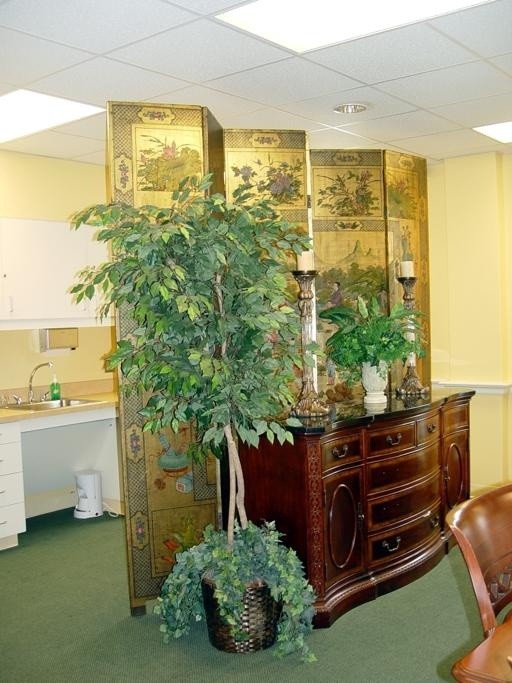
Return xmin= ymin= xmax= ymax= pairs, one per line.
xmin=400 ymin=260 xmax=414 ymax=278
xmin=297 ymin=249 xmax=314 ymax=271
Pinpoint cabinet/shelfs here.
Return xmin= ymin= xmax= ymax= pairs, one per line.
xmin=219 ymin=390 xmax=476 ymax=629
xmin=0 ymin=421 xmax=27 ymax=551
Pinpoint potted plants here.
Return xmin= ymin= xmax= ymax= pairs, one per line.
xmin=319 ymin=294 xmax=426 ymax=412
xmin=69 ymin=169 xmax=321 ymax=670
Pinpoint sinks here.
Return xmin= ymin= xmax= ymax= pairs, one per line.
xmin=9 ymin=398 xmax=104 ymax=410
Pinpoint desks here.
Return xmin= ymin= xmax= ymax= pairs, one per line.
xmin=451 ymin=620 xmax=512 ymax=683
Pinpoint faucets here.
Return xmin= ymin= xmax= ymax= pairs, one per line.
xmin=27 ymin=360 xmax=57 ymax=404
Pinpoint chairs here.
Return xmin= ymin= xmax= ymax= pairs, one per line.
xmin=443 ymin=481 xmax=512 ymax=635
